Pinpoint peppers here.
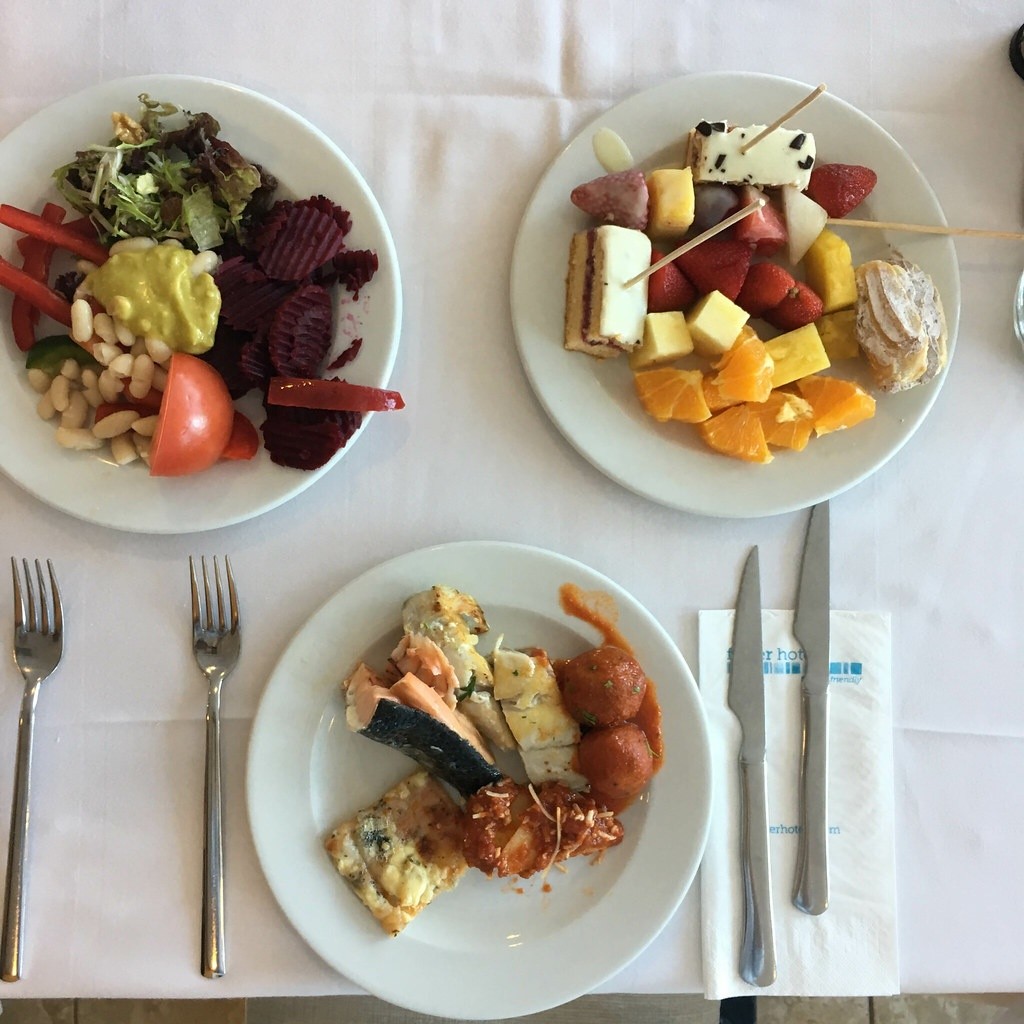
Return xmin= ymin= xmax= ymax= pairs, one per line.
xmin=0 ymin=202 xmax=112 ymax=373
xmin=267 ymin=376 xmax=405 ymax=411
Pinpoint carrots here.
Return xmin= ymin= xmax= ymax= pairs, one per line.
xmin=95 ymin=405 xmax=258 ymax=460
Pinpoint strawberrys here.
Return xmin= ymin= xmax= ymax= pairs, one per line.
xmin=571 ymin=163 xmax=878 ymax=329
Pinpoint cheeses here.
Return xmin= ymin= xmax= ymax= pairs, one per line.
xmin=686 ymin=290 xmax=751 ymax=358
xmin=630 ymin=310 xmax=695 ymax=368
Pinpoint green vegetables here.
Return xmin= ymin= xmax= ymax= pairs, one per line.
xmin=53 ymin=94 xmax=277 ymax=252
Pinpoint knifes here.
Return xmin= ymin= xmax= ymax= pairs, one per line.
xmin=791 ymin=501 xmax=830 ymax=916
xmin=726 ymin=542 xmax=779 ymax=988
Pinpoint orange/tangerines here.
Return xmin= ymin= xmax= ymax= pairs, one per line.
xmin=635 ymin=336 xmax=876 ymax=464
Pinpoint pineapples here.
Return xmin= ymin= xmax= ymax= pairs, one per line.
xmin=763 ymin=233 xmax=860 ymax=391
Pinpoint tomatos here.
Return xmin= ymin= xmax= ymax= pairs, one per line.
xmin=151 ymin=352 xmax=233 ymax=476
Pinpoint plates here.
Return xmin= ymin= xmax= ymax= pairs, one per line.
xmin=1 ymin=72 xmax=404 ymax=536
xmin=508 ymin=70 xmax=962 ymax=519
xmin=246 ymin=540 xmax=716 ymax=1020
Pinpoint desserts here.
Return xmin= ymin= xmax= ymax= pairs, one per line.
xmin=687 ymin=120 xmax=816 ymax=190
xmin=853 ymin=259 xmax=949 ymax=393
xmin=565 ymin=226 xmax=653 ymax=358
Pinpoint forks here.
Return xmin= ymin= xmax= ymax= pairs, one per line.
xmin=0 ymin=555 xmax=63 ymax=982
xmin=190 ymin=553 xmax=244 ymax=980
